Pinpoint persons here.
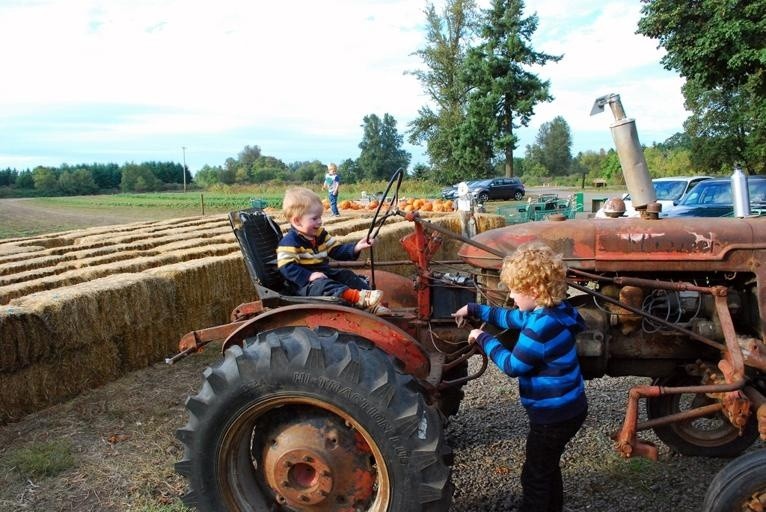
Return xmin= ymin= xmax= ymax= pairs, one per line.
xmin=322 ymin=162 xmax=340 ymax=217
xmin=274 ymin=187 xmax=392 ymax=317
xmin=453 ymin=183 xmax=479 ymax=240
xmin=451 ymin=240 xmax=588 ymax=512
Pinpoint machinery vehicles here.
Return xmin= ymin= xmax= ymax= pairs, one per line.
xmin=166 ymin=94 xmax=766 ymax=512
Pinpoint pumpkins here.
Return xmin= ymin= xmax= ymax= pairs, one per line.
xmin=257 ymin=192 xmax=457 ymax=214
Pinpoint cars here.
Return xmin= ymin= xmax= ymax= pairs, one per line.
xmin=594 ymin=176 xmax=710 ymax=218
xmin=662 ymin=177 xmax=765 ymax=217
xmin=441 ymin=180 xmax=476 ymax=197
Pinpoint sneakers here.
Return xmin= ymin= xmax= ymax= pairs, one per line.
xmin=363 ymin=304 xmax=394 ymax=317
xmin=356 ymin=290 xmax=383 ymax=308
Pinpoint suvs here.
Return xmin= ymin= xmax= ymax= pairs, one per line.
xmin=470 ymin=178 xmax=525 ymax=202
